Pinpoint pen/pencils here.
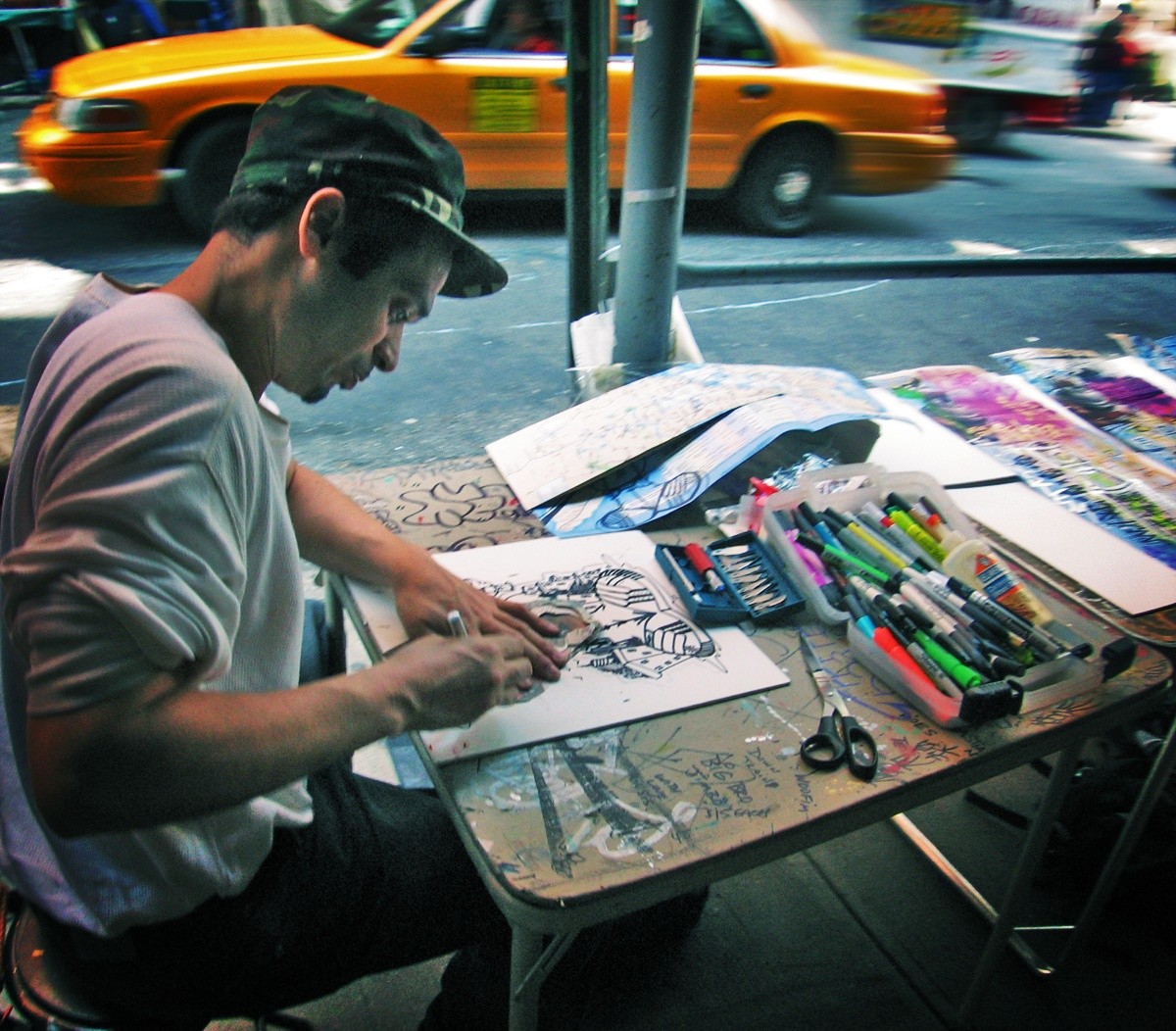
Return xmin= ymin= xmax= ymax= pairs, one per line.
xmin=449 ymin=609 xmax=470 ymax=642
xmin=772 ymin=483 xmax=1096 ymax=718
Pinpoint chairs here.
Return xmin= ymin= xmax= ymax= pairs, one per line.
xmin=0 ymin=713 xmax=359 ymax=1031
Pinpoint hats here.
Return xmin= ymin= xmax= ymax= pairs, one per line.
xmin=229 ymin=87 xmax=510 ymax=299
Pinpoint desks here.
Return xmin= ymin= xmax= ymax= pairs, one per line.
xmin=963 ymin=513 xmax=1176 ymax=984
xmin=319 ymin=450 xmax=1176 ymax=1031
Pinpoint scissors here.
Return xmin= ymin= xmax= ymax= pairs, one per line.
xmin=798 ymin=629 xmax=880 ymax=779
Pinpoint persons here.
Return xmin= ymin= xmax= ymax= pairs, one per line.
xmin=500 ymin=0 xmax=557 ymax=56
xmin=0 ymin=85 xmax=709 ymax=1030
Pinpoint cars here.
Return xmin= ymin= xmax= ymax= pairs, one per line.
xmin=6 ymin=0 xmax=1176 ymax=242
